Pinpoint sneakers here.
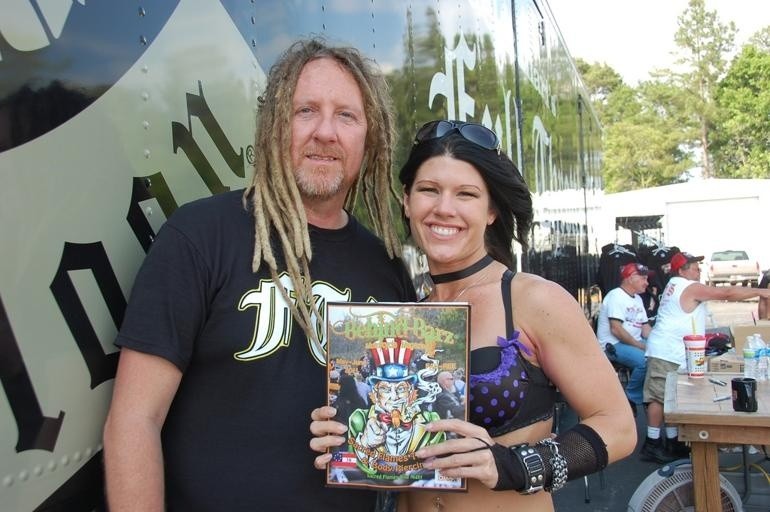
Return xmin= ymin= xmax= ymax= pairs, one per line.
xmin=639 ymin=436 xmax=676 ymax=463
xmin=664 ymin=437 xmax=693 ymax=459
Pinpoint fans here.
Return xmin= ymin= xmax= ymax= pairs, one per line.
xmin=626 ymin=459 xmax=743 ymax=511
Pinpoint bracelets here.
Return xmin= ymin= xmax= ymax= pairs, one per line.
xmin=508 ymin=438 xmax=546 ymax=496
xmin=538 ymin=438 xmax=568 ymax=491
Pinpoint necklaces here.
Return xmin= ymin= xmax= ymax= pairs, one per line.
xmin=426 ymin=253 xmax=494 ymax=287
xmin=428 ymin=263 xmax=498 ymax=302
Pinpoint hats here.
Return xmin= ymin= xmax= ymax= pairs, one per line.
xmin=620 ymin=263 xmax=656 ymax=279
xmin=671 ymin=253 xmax=704 ymax=272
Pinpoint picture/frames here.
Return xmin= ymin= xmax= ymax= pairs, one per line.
xmin=325 ymin=300 xmax=471 ymax=492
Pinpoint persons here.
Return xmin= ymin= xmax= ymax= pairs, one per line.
xmin=329 ymin=353 xmax=465 ymax=490
xmin=100 ymin=32 xmax=417 ymax=510
xmin=307 ymin=120 xmax=639 ymax=510
xmin=641 ymin=250 xmax=770 ymax=465
xmin=758 ymin=268 xmax=770 ymax=321
xmin=598 ymin=262 xmax=655 ymax=416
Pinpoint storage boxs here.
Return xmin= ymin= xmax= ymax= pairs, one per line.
xmin=711 ymin=350 xmax=746 ymax=374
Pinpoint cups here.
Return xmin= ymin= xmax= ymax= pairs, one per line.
xmin=683 ymin=334 xmax=708 ymax=379
xmin=732 ymin=378 xmax=759 ymax=413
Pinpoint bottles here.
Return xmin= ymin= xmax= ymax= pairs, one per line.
xmin=751 ymin=332 xmax=767 ymax=382
xmin=742 ymin=336 xmax=757 ymax=380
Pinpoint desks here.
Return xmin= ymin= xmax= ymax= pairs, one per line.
xmin=664 ymin=368 xmax=770 ymax=512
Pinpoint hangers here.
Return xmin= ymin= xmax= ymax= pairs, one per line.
xmin=608 ymin=225 xmax=673 ymax=256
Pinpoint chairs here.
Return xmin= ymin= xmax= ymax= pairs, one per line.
xmin=579 ymin=314 xmax=638 ymax=416
xmin=552 ymin=391 xmax=608 ymax=504
xmin=729 ymin=323 xmax=770 ymax=371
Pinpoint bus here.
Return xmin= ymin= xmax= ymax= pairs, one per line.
xmin=1 ymin=0 xmax=604 ymax=512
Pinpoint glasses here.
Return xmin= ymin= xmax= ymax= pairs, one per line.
xmin=412 ymin=121 xmax=502 ymax=157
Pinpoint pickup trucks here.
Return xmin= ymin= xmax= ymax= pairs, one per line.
xmin=705 ymin=250 xmax=761 ymax=288
